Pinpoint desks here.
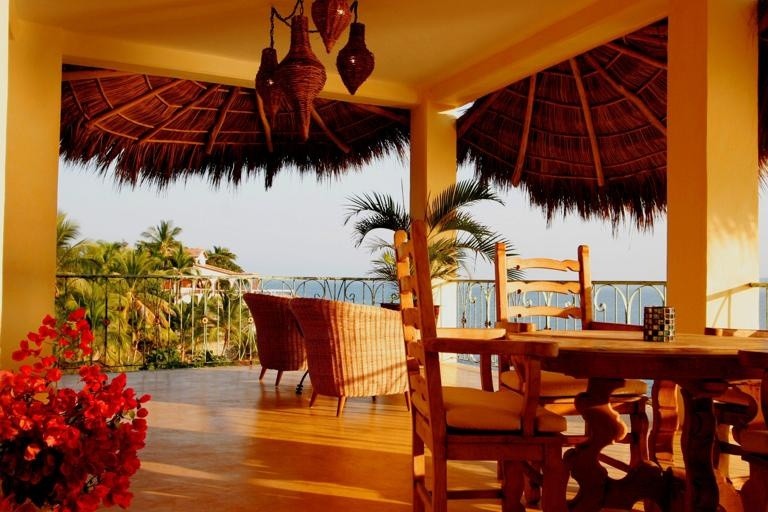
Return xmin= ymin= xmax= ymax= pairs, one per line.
xmin=511 ymin=326 xmax=767 ymax=508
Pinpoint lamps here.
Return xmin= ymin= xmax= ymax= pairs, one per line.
xmin=254 ymin=0 xmax=378 ymax=158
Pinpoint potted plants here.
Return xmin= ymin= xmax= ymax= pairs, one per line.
xmin=342 ymin=174 xmax=532 ymax=328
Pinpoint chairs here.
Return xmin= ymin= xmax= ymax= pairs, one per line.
xmin=241 ymin=292 xmax=309 ymax=387
xmin=392 ymin=216 xmax=568 ymax=512
xmin=288 ymin=294 xmax=411 ymax=417
xmin=492 ymin=242 xmax=650 ymax=504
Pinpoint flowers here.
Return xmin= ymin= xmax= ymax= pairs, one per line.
xmin=0 ymin=302 xmax=154 ymax=511
